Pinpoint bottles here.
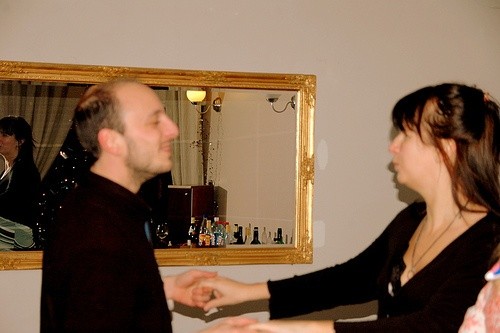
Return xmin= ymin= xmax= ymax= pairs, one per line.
xmin=188 ymin=216 xmax=293 ymax=247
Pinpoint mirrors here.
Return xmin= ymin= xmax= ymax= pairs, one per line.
xmin=0 ymin=60 xmax=317 ymax=270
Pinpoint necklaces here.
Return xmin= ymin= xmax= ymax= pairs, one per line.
xmin=407 ymin=200 xmax=469 ymax=279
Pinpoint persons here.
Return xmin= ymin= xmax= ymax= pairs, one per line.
xmin=184 ymin=83 xmax=499 ymax=333
xmin=459 ymin=241 xmax=500 ymax=333
xmin=40 ymin=79 xmax=260 ymax=333
xmin=0 ymin=115 xmax=41 ymax=251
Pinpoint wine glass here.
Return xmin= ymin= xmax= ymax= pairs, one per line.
xmin=156 ymin=223 xmax=168 ymax=249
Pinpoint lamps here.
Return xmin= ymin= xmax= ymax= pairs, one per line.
xmin=187 ymin=90 xmax=225 ymax=114
xmin=266 ymin=93 xmax=296 ymax=113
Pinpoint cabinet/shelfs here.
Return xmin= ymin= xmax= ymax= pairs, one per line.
xmin=166 ymin=184 xmax=215 ymax=245
